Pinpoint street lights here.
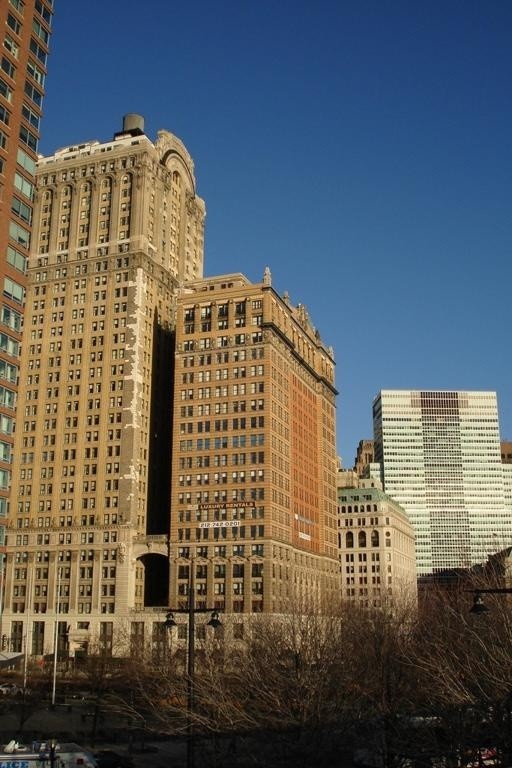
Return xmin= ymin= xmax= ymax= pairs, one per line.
xmin=464 ymin=586 xmax=512 ymax=614
xmin=162 ymin=586 xmax=221 ymax=768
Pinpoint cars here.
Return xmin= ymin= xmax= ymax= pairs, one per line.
xmin=0 ymin=683 xmax=32 ymax=696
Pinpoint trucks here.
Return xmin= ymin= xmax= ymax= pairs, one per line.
xmin=0 ymin=741 xmax=98 ymax=768
